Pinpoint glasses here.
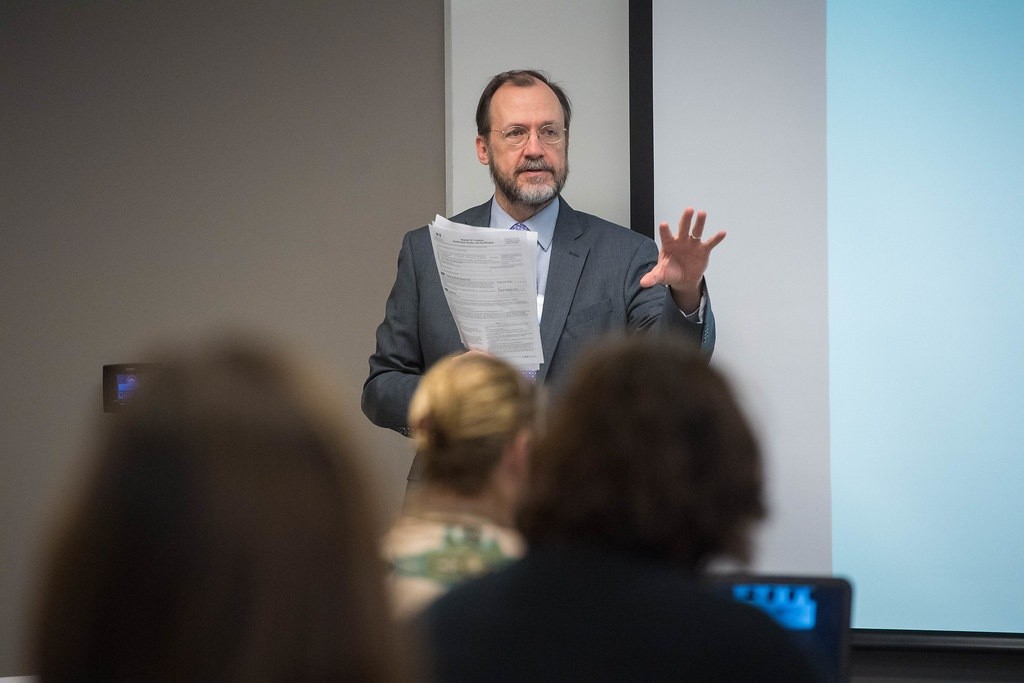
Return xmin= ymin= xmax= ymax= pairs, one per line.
xmin=486 ymin=124 xmax=571 ymax=146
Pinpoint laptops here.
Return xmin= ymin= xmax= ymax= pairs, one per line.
xmin=724 ymin=571 xmax=854 ymax=678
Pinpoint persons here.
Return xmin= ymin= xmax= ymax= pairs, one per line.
xmin=361 ymin=57 xmax=727 ymax=518
xmin=400 ymin=329 xmax=836 ymax=683
xmin=20 ymin=344 xmax=416 ymax=683
xmin=378 ymin=350 xmax=530 ymax=620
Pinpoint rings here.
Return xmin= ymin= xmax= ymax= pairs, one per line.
xmin=692 ymin=232 xmax=701 ymax=240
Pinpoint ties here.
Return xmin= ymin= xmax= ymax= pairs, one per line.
xmin=510 ymin=223 xmax=536 ymax=389
